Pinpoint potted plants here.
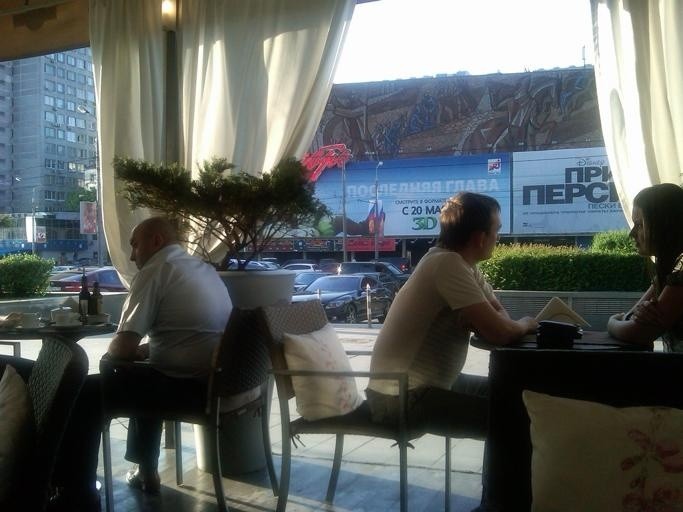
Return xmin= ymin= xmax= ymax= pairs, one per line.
xmin=110 ymin=153 xmax=335 ymax=475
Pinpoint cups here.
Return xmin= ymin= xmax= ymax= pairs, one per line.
xmin=18 ymin=308 xmax=79 ymax=324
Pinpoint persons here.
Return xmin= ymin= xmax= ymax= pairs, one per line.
xmin=42 ymin=217 xmax=234 ymax=512
xmin=365 ymin=192 xmax=540 ymax=512
xmin=608 ymin=183 xmax=683 ymax=344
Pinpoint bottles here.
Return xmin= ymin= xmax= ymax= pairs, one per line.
xmin=80 ymin=270 xmax=101 ymax=319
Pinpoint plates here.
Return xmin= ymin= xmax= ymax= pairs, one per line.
xmin=13 ymin=323 xmax=82 ymax=332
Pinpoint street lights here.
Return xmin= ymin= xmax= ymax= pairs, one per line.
xmin=373 ymin=159 xmax=386 ymax=259
xmin=15 ymin=176 xmax=36 ymax=256
xmin=77 ymin=105 xmax=103 ymax=266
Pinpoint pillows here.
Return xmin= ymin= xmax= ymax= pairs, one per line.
xmin=0 ymin=364 xmax=33 ymax=500
xmin=206 ymin=329 xmax=263 ymax=416
xmin=283 ymin=322 xmax=364 ymax=422
xmin=522 ymin=390 xmax=682 ymax=511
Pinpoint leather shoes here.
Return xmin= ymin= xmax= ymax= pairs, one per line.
xmin=126 ymin=465 xmax=162 ymax=492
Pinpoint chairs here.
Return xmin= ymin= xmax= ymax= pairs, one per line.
xmin=101 ymin=300 xmax=292 ymax=512
xmin=0 ymin=334 xmax=89 ymax=487
xmin=261 ymin=300 xmax=452 ymax=511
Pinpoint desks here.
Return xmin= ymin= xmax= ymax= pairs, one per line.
xmin=470 ymin=331 xmax=682 ymax=512
xmin=0 ymin=303 xmax=119 ymax=340
